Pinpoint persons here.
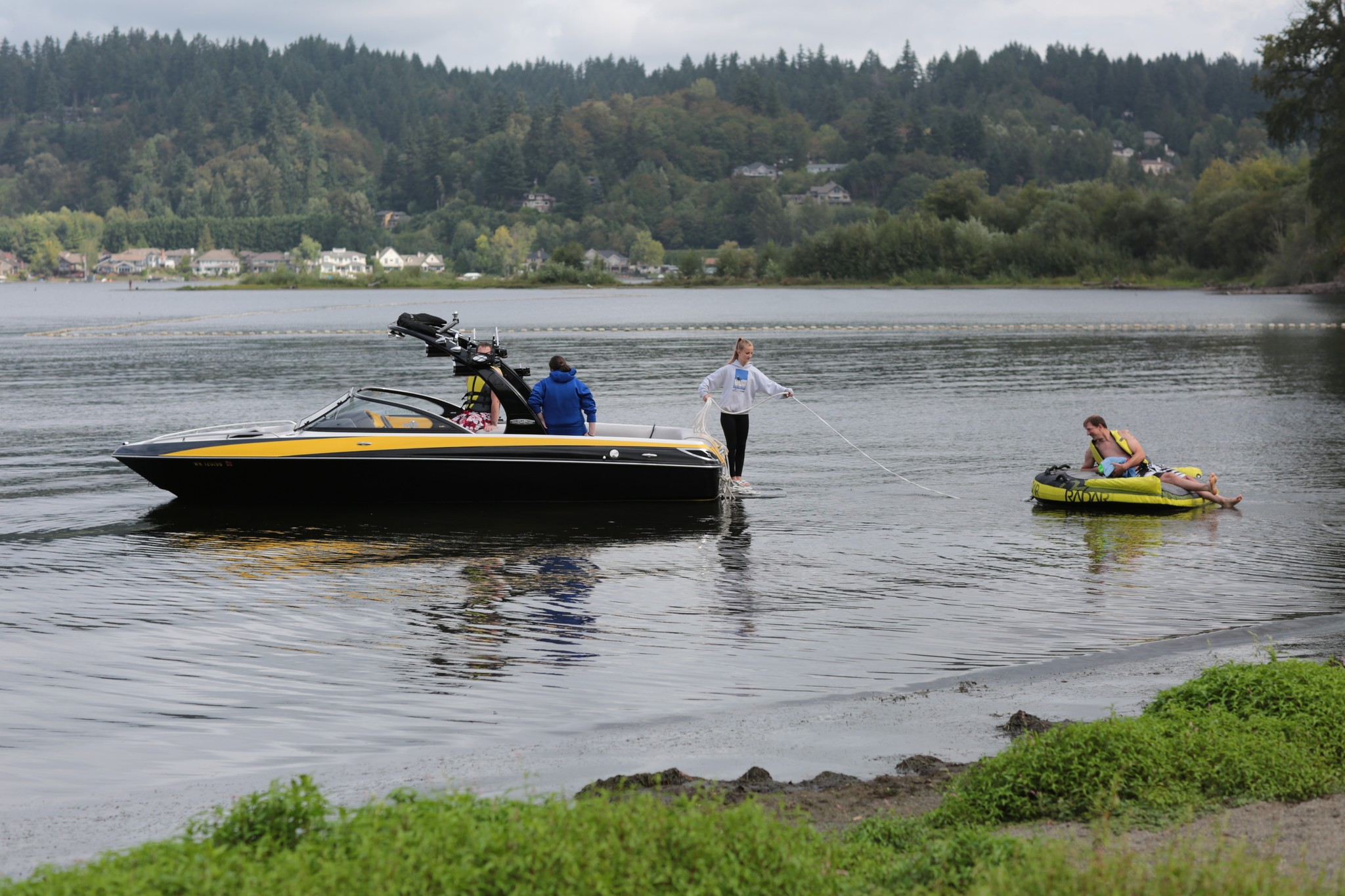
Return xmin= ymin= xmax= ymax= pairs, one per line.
xmin=1081 ymin=415 xmax=1244 ymax=508
xmin=450 ymin=342 xmax=504 ymax=433
xmin=527 ymin=356 xmax=597 ymax=436
xmin=698 ymin=337 xmax=794 ymax=487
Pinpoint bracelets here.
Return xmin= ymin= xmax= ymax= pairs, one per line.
xmin=588 ymin=432 xmax=595 ymax=436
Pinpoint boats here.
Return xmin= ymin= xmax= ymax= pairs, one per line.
xmin=1031 ymin=464 xmax=1219 ymax=515
xmin=111 ymin=311 xmax=730 ymax=522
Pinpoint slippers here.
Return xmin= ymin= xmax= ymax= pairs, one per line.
xmin=730 ymin=480 xmax=751 ymax=489
